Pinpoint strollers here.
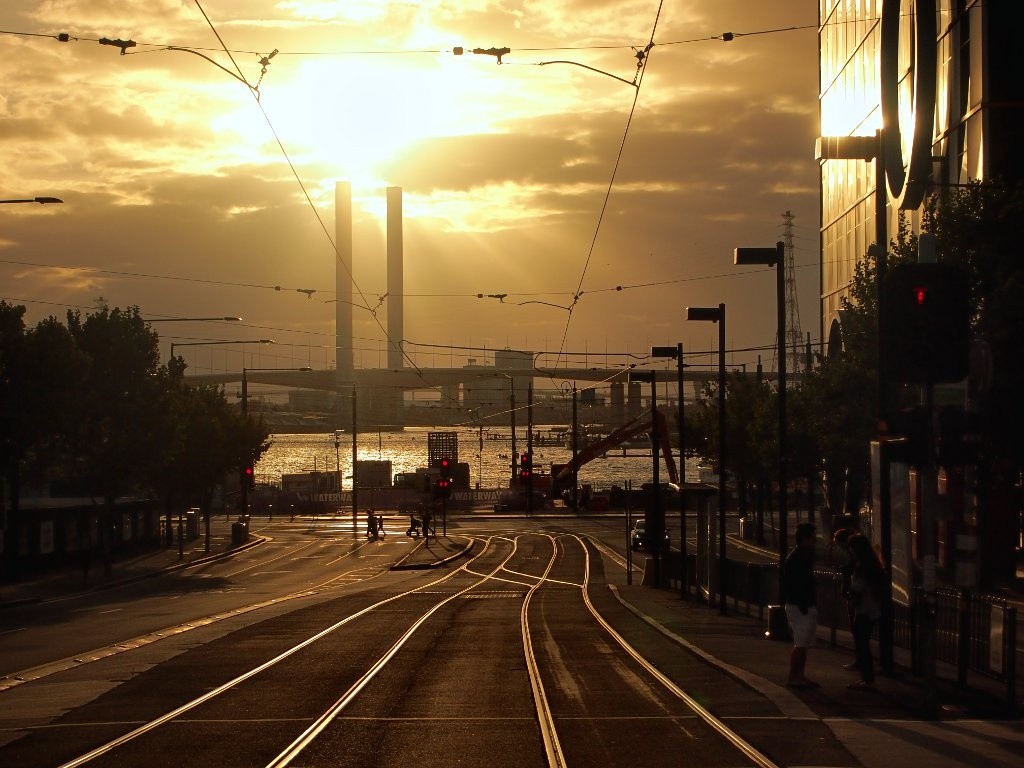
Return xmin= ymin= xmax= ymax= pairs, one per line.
xmin=406 ymin=517 xmax=421 ymax=536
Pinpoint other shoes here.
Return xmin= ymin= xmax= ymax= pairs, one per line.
xmin=803 ymin=678 xmax=820 ymax=690
xmin=785 ymin=680 xmax=808 ymax=690
xmin=846 ymin=679 xmax=871 ymax=692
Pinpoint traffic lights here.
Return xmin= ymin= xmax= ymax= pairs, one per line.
xmin=246 ymin=468 xmax=255 ymax=492
xmin=438 ymin=480 xmax=443 ymax=494
xmin=521 ymin=471 xmax=529 ymax=480
xmin=522 ymin=456 xmax=528 ymax=468
xmin=442 ymin=459 xmax=448 ymax=477
xmin=443 ymin=480 xmax=449 ymax=494
xmin=901 ymin=264 xmax=938 ymax=377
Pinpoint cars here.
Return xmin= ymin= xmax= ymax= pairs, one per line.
xmin=631 ymin=518 xmax=672 ymax=554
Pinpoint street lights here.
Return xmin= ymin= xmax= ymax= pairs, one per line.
xmin=906 ymin=179 xmax=1007 ymax=190
xmin=141 ymin=317 xmax=241 ymax=323
xmin=735 ymin=241 xmax=794 ymax=643
xmin=0 ymin=196 xmax=63 ymax=204
xmin=170 ymin=339 xmax=277 ymax=359
xmin=674 ymin=363 xmax=746 ymax=376
xmin=236 ymin=366 xmax=313 ymax=541
xmin=814 ymin=129 xmax=887 ymax=680
xmin=650 ymin=342 xmax=690 ymax=607
xmin=685 ymin=304 xmax=729 ymax=616
xmin=628 ymin=369 xmax=663 ymax=592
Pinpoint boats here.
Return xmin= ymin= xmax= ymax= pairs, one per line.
xmin=607 ymin=428 xmax=653 ymax=449
xmin=564 ymin=424 xmax=594 ymax=450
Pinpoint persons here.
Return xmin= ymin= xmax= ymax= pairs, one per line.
xmin=366 ymin=509 xmax=375 ymax=537
xmin=834 ymin=529 xmax=872 ymax=673
xmin=406 ymin=514 xmax=421 ymax=536
xmin=377 ymin=515 xmax=386 ymax=536
xmin=778 ymin=522 xmax=824 ymax=690
xmin=845 ymin=533 xmax=886 ymax=690
xmin=420 ymin=505 xmax=434 ymax=536
